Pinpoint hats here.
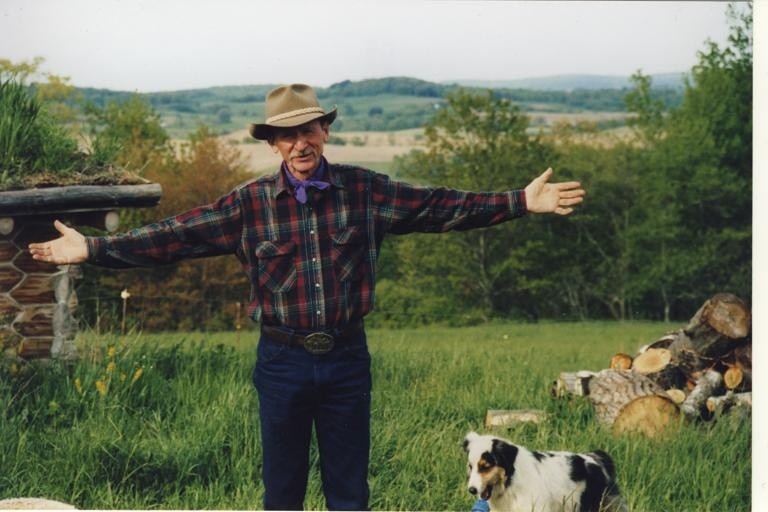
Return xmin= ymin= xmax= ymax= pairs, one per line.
xmin=248 ymin=83 xmax=338 ymax=140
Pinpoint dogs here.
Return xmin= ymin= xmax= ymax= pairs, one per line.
xmin=462 ymin=430 xmax=628 ymax=512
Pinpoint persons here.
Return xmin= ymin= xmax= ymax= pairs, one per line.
xmin=26 ymin=105 xmax=585 ymax=511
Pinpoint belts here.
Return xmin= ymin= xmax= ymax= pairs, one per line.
xmin=261 ymin=321 xmax=363 ymax=352
